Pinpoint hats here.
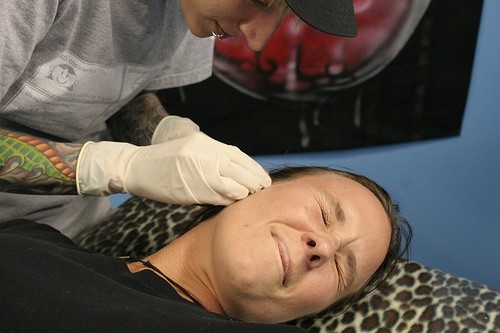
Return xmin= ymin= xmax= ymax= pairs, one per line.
xmin=284 ymin=0 xmax=357 ymax=37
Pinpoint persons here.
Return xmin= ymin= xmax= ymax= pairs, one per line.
xmin=2 ymin=2 xmax=357 ymax=238
xmin=1 ymin=166 xmax=412 ymax=333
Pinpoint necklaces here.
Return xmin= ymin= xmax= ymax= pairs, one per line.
xmin=113 ymin=253 xmax=235 ymax=320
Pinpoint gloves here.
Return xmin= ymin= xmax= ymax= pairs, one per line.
xmin=149 ymin=115 xmax=200 ymax=147
xmin=75 ymin=131 xmax=272 ymax=208
